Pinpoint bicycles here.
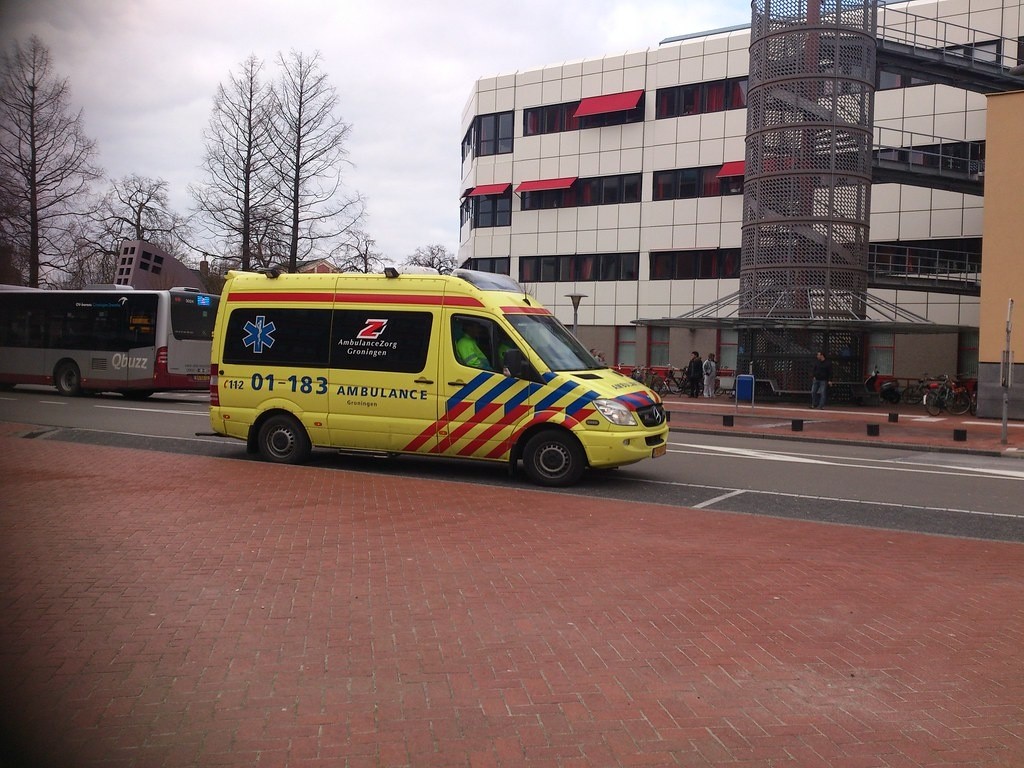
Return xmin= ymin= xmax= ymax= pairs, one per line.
xmin=900 ymin=372 xmax=976 ymax=416
xmin=618 ymin=361 xmax=729 ymax=398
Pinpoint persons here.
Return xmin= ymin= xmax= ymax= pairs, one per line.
xmin=686 ymin=351 xmax=703 ymax=398
xmin=454 ymin=317 xmax=518 ymax=374
xmin=809 ymin=350 xmax=833 ymax=410
xmin=702 ymin=353 xmax=718 ymax=399
xmin=590 ymin=348 xmax=607 ymax=369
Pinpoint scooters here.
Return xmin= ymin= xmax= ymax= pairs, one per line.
xmin=866 ymin=366 xmax=902 ymax=403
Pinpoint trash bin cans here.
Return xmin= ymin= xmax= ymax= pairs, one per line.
xmin=737 ymin=375 xmax=753 ymax=401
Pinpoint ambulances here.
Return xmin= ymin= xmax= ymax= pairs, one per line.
xmin=209 ymin=266 xmax=672 ymax=490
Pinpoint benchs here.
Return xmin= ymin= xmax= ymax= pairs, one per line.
xmin=850 ymin=385 xmax=879 ymax=408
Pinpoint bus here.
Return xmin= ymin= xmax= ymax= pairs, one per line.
xmin=0 ymin=282 xmax=222 ymax=399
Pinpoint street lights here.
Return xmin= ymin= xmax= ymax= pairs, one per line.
xmin=563 ymin=293 xmax=589 ymax=338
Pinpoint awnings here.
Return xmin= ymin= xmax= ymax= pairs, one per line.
xmin=572 ymin=88 xmax=644 ymax=118
xmin=514 ymin=176 xmax=578 ymax=193
xmin=458 ymin=182 xmax=512 ymax=201
xmin=716 ymin=160 xmax=745 ymax=179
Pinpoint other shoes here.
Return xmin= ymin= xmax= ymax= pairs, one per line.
xmin=710 ymin=395 xmax=717 ymax=398
xmin=811 ymin=406 xmax=815 ymax=409
xmin=694 ymin=395 xmax=698 ymax=398
xmin=820 ymin=406 xmax=824 ymax=410
xmin=704 ymin=395 xmax=710 ymax=398
xmin=688 ymin=395 xmax=694 ymax=398
